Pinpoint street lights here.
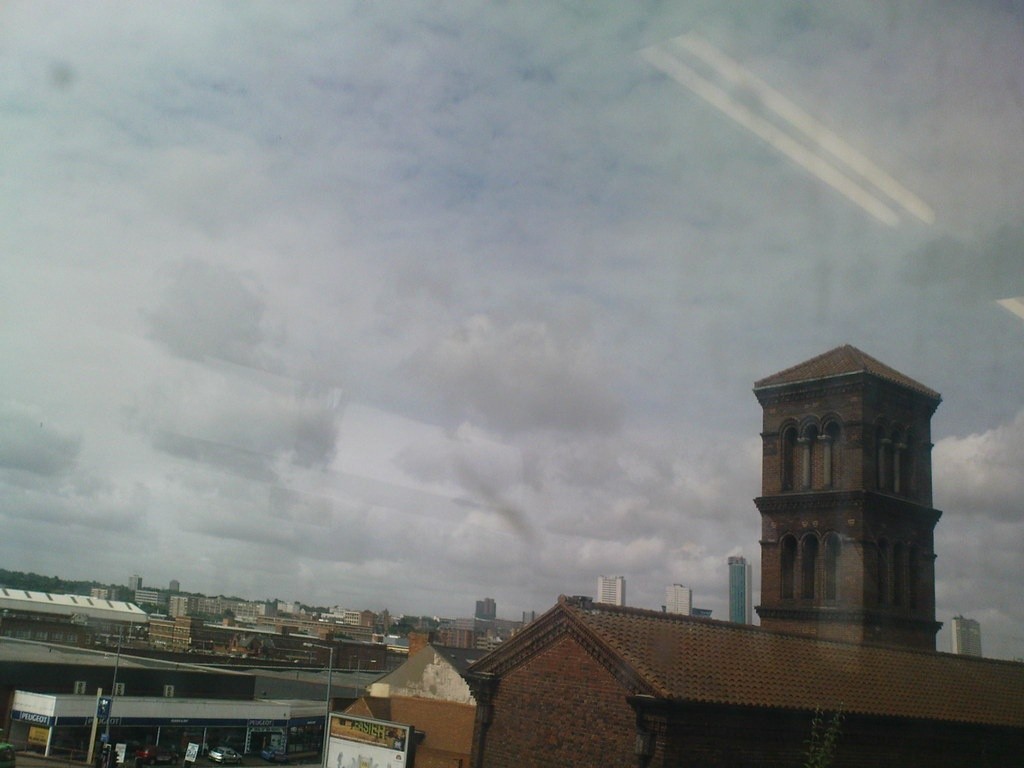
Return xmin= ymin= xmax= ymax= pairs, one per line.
xmin=102 ymin=625 xmax=142 ymax=742
xmin=303 ymin=641 xmax=333 ymax=767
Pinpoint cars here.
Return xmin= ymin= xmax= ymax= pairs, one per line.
xmin=137 ymin=744 xmax=178 ymax=765
xmin=208 ymin=746 xmax=243 ymax=765
xmin=260 ymin=746 xmax=288 ymax=763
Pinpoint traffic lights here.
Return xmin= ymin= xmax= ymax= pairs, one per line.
xmin=100 ymin=744 xmax=112 ymax=768
xmin=108 ymin=751 xmax=120 ymax=768
